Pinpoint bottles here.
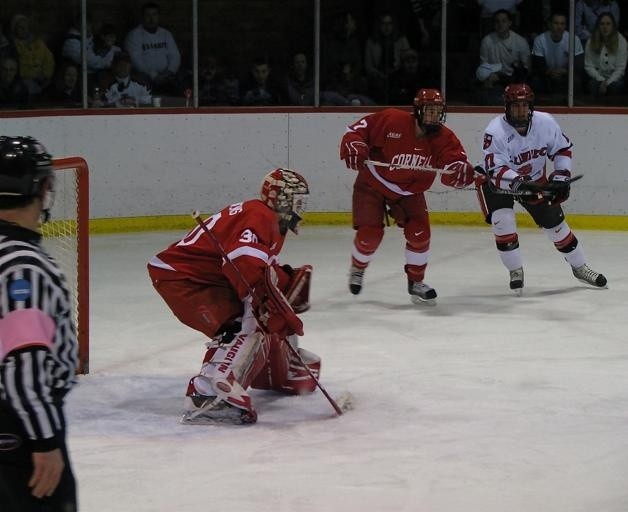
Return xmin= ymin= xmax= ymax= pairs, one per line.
xmin=183 ymin=89 xmax=192 ymax=108
xmin=93 ymin=88 xmax=99 ymax=108
xmin=295 ymin=90 xmax=309 ymax=106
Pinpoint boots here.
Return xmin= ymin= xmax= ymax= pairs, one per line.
xmin=565 ymin=256 xmax=607 ymax=286
xmin=510 ymin=267 xmax=523 ymax=288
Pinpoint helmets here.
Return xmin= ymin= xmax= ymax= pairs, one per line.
xmin=414 ymin=89 xmax=448 ymax=135
xmin=503 ymin=84 xmax=534 ymax=127
xmin=0 ymin=134 xmax=52 ymax=228
xmin=260 ymin=168 xmax=308 ymax=233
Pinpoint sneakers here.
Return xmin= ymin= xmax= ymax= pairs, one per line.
xmin=350 ymin=265 xmax=364 ymax=294
xmin=184 ymin=390 xmax=257 ymax=424
xmin=408 ymin=278 xmax=437 ymax=299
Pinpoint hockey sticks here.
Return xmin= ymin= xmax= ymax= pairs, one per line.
xmin=363 ymin=160 xmax=584 ymax=192
xmin=474 ymin=164 xmax=553 ymax=196
xmin=190 ymin=209 xmax=358 ymax=415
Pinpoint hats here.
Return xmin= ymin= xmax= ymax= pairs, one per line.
xmin=476 ymin=63 xmax=503 ymax=81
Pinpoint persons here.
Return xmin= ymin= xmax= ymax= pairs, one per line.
xmin=341 ymin=90 xmax=473 ymax=300
xmin=2 ymin=2 xmax=626 ymax=108
xmin=0 ymin=138 xmax=81 ymax=511
xmin=147 ymin=167 xmax=309 ymax=422
xmin=483 ymin=82 xmax=607 ymax=288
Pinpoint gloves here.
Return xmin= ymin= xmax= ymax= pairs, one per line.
xmin=342 ymin=141 xmax=369 ymax=170
xmin=257 ymin=299 xmax=285 ymax=333
xmin=510 ymin=175 xmax=544 ymax=193
xmin=441 ymin=162 xmax=474 ymax=187
xmin=543 ymin=173 xmax=570 ymax=206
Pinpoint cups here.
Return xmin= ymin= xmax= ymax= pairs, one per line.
xmin=152 ymin=98 xmax=161 ymax=107
